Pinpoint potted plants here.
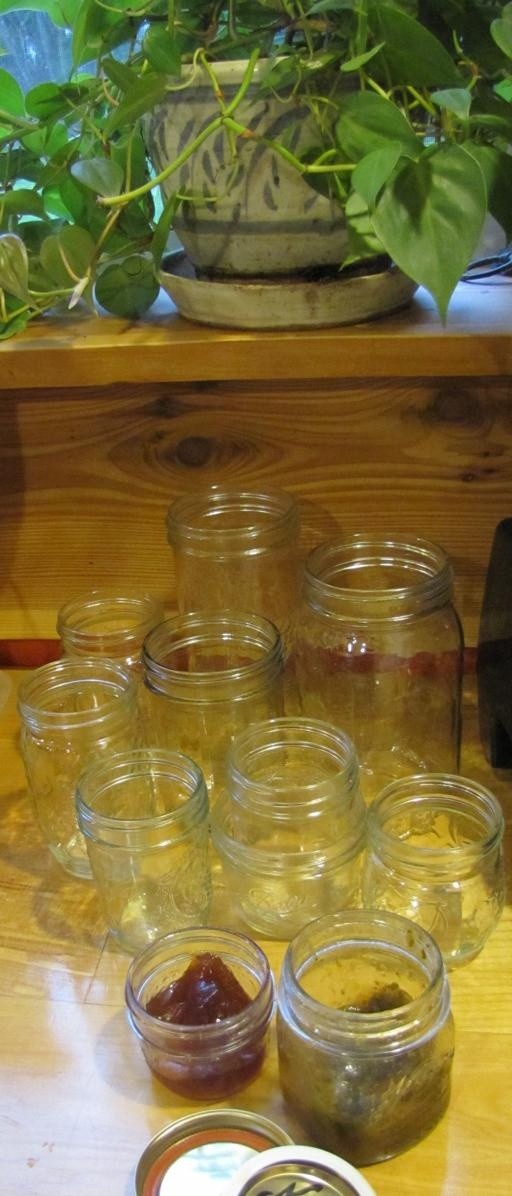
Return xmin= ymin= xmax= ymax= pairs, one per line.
xmin=0 ymin=2 xmax=511 ymax=338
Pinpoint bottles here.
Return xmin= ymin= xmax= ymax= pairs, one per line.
xmin=123 ymin=929 xmax=270 ymax=1098
xmin=268 ymin=909 xmax=455 ymax=1167
xmin=208 ymin=722 xmax=369 ymax=944
xmin=14 ymin=655 xmax=152 ymax=880
xmin=73 ymin=744 xmax=210 ymax=958
xmin=54 ymin=588 xmax=145 ymax=678
xmin=357 ymin=776 xmax=503 ymax=967
xmin=136 ymin=610 xmax=287 ymax=788
xmin=165 ymin=480 xmax=299 ymax=718
xmin=306 ymin=530 xmax=463 ymax=797
